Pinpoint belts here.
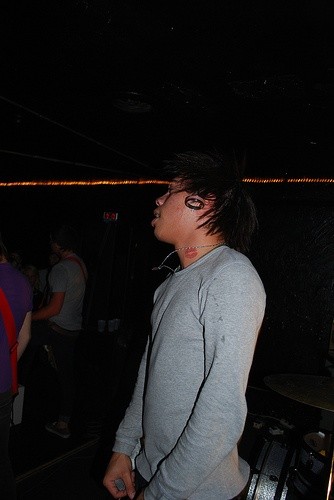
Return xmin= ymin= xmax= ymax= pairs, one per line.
xmin=49 ymin=323 xmax=81 ymax=337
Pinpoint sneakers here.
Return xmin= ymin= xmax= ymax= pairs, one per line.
xmin=45 ymin=421 xmax=70 ymax=438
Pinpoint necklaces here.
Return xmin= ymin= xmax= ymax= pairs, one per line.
xmin=152 ymin=242 xmax=228 ymax=272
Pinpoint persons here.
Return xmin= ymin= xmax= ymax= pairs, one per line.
xmin=103 ymin=152 xmax=266 ymax=500
xmin=0 ymin=223 xmax=89 ymax=500
